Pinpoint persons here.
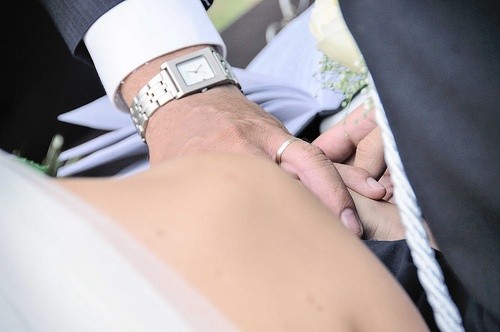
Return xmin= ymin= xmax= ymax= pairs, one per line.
xmin=0 ymin=0 xmax=500 ymax=332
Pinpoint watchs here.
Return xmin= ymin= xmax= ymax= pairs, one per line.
xmin=130 ymin=48 xmax=244 ymax=144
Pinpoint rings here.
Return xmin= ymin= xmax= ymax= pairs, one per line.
xmin=275 ymin=138 xmax=300 ymax=166
